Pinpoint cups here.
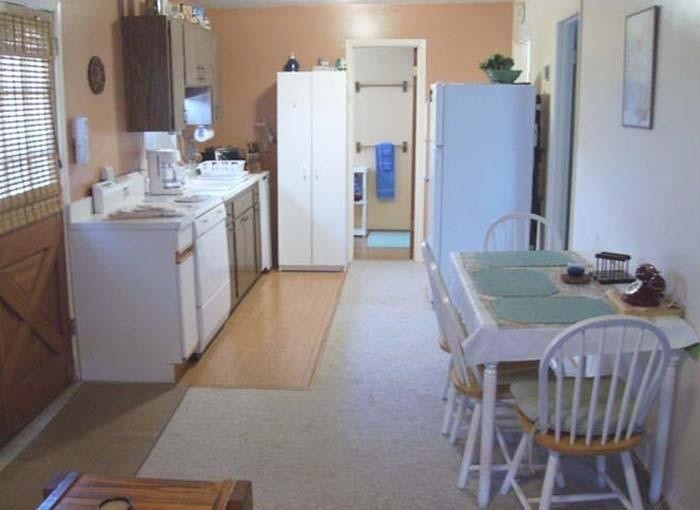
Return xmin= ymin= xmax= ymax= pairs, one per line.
xmin=566 ymin=261 xmax=584 ymax=277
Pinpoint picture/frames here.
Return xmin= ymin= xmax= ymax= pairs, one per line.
xmin=621 ymin=5 xmax=660 ymax=130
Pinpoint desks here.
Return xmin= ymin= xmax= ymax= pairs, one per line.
xmin=36 ymin=469 xmax=251 ymax=509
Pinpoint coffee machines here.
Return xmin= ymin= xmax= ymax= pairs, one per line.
xmin=145 ymin=147 xmax=185 ymax=195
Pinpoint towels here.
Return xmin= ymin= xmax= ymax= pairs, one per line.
xmin=375 ymin=142 xmax=395 ymax=198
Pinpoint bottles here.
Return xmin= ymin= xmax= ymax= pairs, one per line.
xmin=285 ymin=49 xmax=298 ymax=72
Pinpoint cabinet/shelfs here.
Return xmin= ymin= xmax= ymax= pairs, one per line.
xmin=354 ymin=167 xmax=368 ymax=237
xmin=121 ymin=15 xmax=222 ymax=132
xmin=276 ymin=70 xmax=347 ymax=272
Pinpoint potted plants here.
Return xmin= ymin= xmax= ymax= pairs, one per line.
xmin=478 ymin=53 xmax=523 ymax=84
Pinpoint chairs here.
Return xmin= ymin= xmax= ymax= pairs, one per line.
xmin=484 ymin=212 xmax=560 ymax=251
xmin=500 ymin=314 xmax=671 ymax=509
xmin=430 ymin=262 xmax=511 ymax=490
xmin=420 ymin=240 xmax=458 ymax=436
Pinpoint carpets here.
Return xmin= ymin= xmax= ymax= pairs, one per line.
xmin=134 ymin=260 xmax=582 ymax=510
xmin=367 ymin=231 xmax=411 ymax=248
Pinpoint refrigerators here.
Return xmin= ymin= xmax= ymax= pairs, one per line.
xmin=426 ymin=80 xmax=537 ymax=257
